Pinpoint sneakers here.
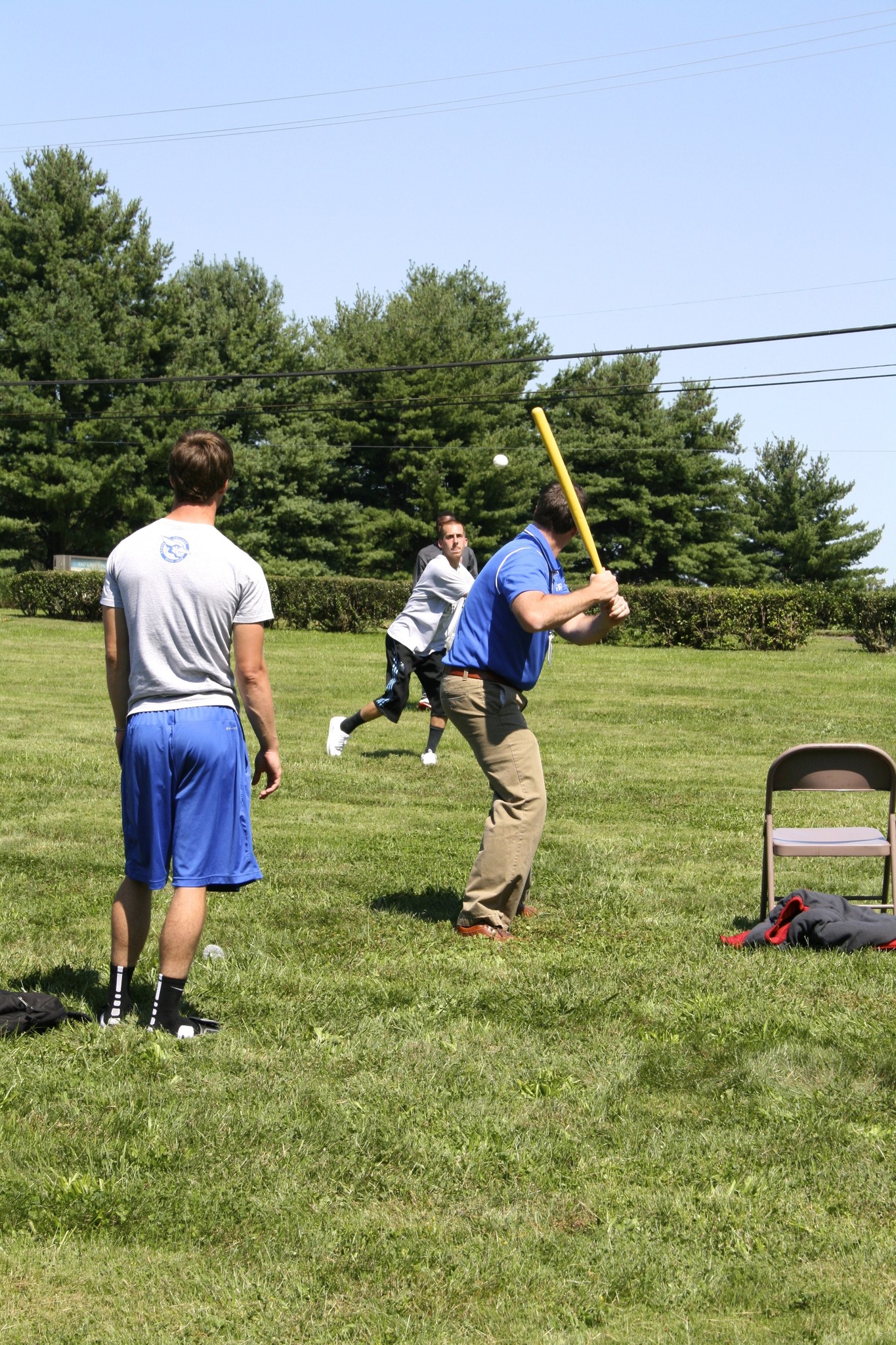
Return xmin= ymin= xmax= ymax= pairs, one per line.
xmin=421 ymin=749 xmax=438 ymax=765
xmin=326 ymin=716 xmax=348 ymax=755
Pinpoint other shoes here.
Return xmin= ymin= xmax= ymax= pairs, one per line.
xmin=419 ymin=693 xmax=432 ymax=710
xmin=516 ymin=903 xmax=537 ymax=919
xmin=457 ymin=923 xmax=530 ymax=945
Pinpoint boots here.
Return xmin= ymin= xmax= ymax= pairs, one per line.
xmin=100 ymin=961 xmax=134 ymax=1029
xmin=147 ymin=973 xmax=220 ymax=1037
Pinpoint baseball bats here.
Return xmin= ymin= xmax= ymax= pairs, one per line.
xmin=532 ymin=405 xmax=604 ymax=576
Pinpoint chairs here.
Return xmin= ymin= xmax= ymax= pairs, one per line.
xmin=761 ymin=743 xmax=896 ymax=923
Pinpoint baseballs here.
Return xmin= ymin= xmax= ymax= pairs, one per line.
xmin=493 ymin=454 xmax=509 ymax=469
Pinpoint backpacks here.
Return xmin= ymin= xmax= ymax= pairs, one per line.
xmin=0 ymin=989 xmax=92 ymax=1038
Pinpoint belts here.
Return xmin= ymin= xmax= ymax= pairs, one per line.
xmin=443 ymin=665 xmax=521 ymax=692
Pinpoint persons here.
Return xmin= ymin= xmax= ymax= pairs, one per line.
xmin=326 ymin=520 xmax=476 ymax=765
xmin=100 ymin=430 xmax=281 ymax=1041
xmin=440 ymin=480 xmax=630 ymax=941
xmin=414 ymin=510 xmax=478 ymax=711
